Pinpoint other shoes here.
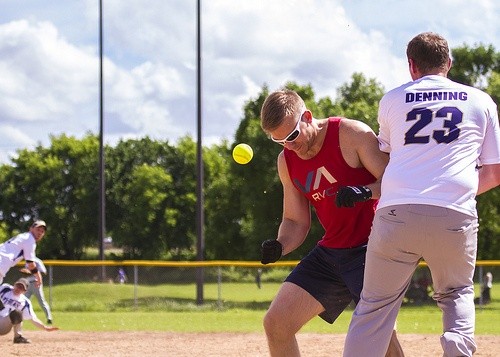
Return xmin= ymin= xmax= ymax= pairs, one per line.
xmin=14 ymin=335 xmax=30 ymax=343
xmin=48 ymin=320 xmax=51 ymax=323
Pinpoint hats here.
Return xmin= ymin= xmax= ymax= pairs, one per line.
xmin=15 ymin=278 xmax=29 ymax=291
xmin=32 ymin=220 xmax=47 ymax=230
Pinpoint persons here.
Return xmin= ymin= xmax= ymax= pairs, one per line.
xmin=403 ymin=274 xmax=428 ymax=305
xmin=342 ymin=31 xmax=500 ymax=357
xmin=0 ymin=278 xmax=60 ymax=344
xmin=20 ymin=256 xmax=53 ymax=325
xmin=481 ymin=270 xmax=493 ymax=304
xmin=116 ymin=267 xmax=128 ymax=284
xmin=260 ymin=90 xmax=406 ymax=357
xmin=0 ymin=219 xmax=47 ymax=286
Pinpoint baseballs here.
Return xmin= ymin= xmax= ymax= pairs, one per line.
xmin=232 ymin=143 xmax=254 ymax=165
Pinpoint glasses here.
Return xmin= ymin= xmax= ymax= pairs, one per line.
xmin=271 ymin=112 xmax=303 ymax=145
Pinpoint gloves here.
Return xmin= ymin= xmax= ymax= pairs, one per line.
xmin=335 ymin=185 xmax=373 ymax=207
xmin=261 ymin=239 xmax=282 ymax=264
